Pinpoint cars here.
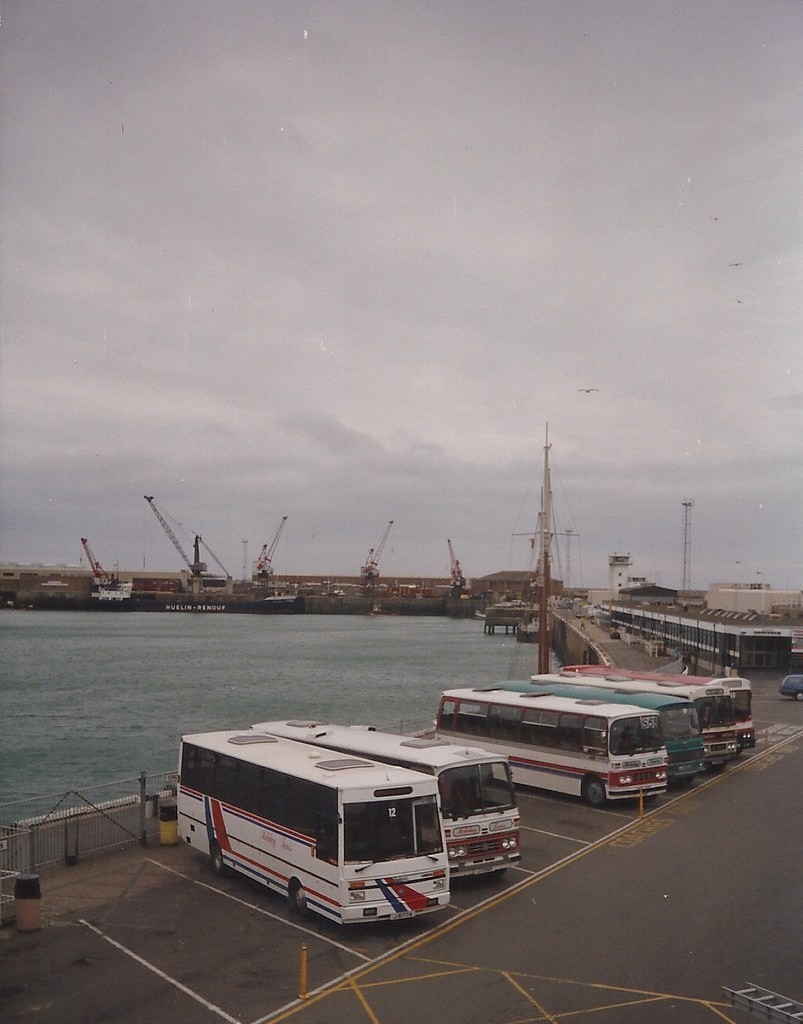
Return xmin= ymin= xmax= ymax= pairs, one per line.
xmin=780 ymin=674 xmax=803 ymax=701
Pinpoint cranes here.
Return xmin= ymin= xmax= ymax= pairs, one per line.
xmin=140 ymin=494 xmax=229 ymax=583
xmin=362 ymin=520 xmax=394 ymax=583
xmin=254 ymin=514 xmax=288 ymax=578
xmin=447 ymin=538 xmax=461 ymax=586
xmin=79 ymin=536 xmax=112 ymax=591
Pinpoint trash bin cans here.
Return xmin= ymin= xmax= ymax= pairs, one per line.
xmin=160 ymin=801 xmax=179 ymax=847
xmin=15 ymin=873 xmax=42 ymax=932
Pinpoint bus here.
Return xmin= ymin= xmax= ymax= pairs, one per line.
xmin=561 ymin=664 xmax=756 ymax=758
xmin=486 ymin=679 xmax=706 ymax=783
xmin=435 ymin=688 xmax=667 ymax=808
xmin=174 ymin=732 xmax=451 ymax=927
xmin=250 ymin=720 xmax=524 ymax=881
xmin=531 ymin=671 xmax=736 ymax=769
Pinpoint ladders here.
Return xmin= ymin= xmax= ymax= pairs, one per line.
xmin=722 ymin=982 xmax=803 ymax=1024
